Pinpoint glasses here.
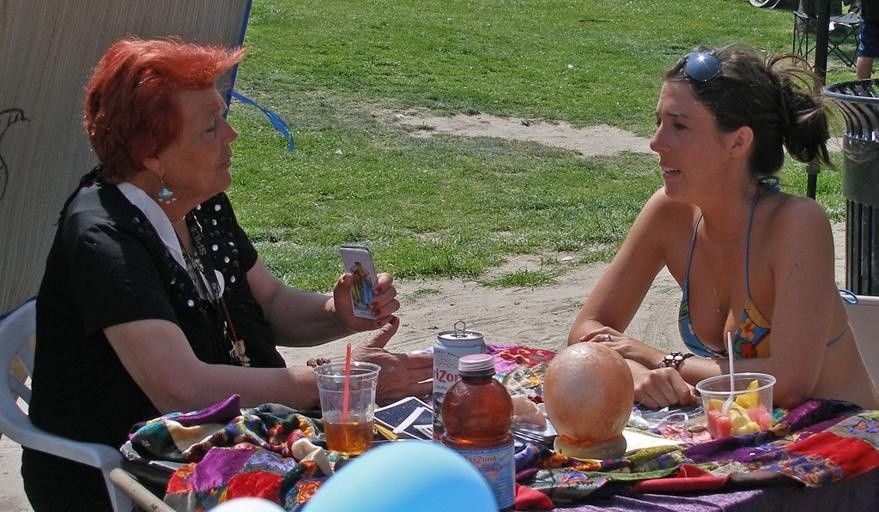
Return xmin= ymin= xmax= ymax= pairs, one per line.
xmin=681 ymin=48 xmax=760 ymax=123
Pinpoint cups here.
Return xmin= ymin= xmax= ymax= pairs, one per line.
xmin=312 ymin=361 xmax=381 ymax=453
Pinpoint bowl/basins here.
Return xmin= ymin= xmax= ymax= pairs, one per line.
xmin=697 ymin=372 xmax=776 ymax=439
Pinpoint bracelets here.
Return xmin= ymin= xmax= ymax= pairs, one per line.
xmin=307 ymin=357 xmax=338 ymax=407
xmin=657 ymin=352 xmax=696 ymax=371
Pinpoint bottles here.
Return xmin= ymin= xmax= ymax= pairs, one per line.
xmin=440 ymin=351 xmax=516 ymax=512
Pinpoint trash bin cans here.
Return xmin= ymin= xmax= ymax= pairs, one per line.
xmin=823 ymin=77 xmax=879 ymax=296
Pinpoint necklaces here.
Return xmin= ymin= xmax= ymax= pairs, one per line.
xmin=142 ymin=188 xmax=251 ymax=367
xmin=700 ymin=207 xmax=748 ymax=315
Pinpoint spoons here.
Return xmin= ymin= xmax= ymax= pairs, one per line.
xmin=721 ymin=329 xmax=735 ymax=417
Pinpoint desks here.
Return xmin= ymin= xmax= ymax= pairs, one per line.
xmin=119 ymin=343 xmax=878 ymax=511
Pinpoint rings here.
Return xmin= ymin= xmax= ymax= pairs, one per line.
xmin=604 ymin=333 xmax=610 ymax=342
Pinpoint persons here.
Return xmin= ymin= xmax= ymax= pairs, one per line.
xmin=568 ymin=41 xmax=879 ymax=411
xmin=21 ymin=38 xmax=434 ymax=512
xmin=855 ymin=1 xmax=879 ymax=80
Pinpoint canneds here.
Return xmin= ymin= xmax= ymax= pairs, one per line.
xmin=433 ymin=320 xmax=484 ymax=441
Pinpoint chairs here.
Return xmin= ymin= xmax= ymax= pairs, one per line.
xmin=0 ymin=296 xmax=137 ymax=511
xmin=840 ymin=290 xmax=879 ymax=397
